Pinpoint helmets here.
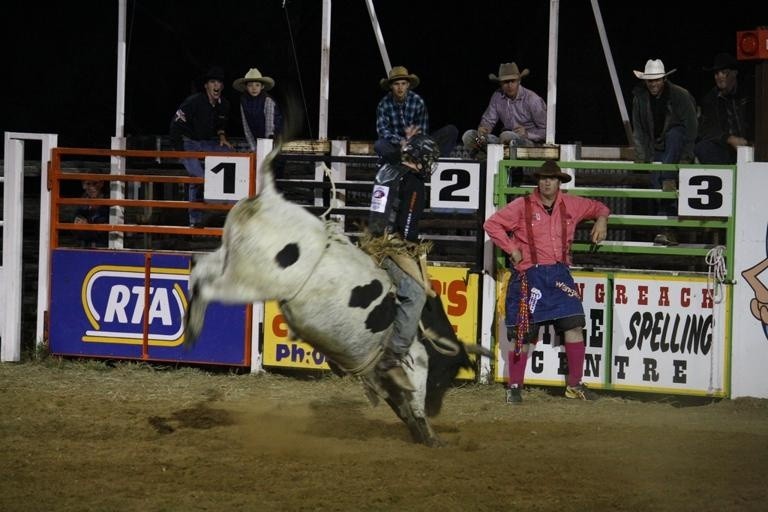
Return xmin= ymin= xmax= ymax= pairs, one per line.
xmin=401 ymin=133 xmax=440 ymax=177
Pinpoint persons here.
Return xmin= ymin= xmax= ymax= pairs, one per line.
xmin=483 ymin=160 xmax=610 ymax=403
xmin=166 ymin=69 xmax=236 ymax=239
xmin=69 ymin=168 xmax=109 ymax=248
xmin=226 ymin=68 xmax=283 ymax=151
xmin=462 ymin=62 xmax=547 ymax=164
xmin=630 ymin=59 xmax=698 ymax=246
xmin=371 ymin=65 xmax=459 ymax=177
xmin=370 ymin=135 xmax=440 ymax=392
xmin=697 ymin=47 xmax=753 ymax=161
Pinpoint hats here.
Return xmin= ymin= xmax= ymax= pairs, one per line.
xmin=534 ymin=161 xmax=571 ymax=183
xmin=380 ymin=66 xmax=419 ymax=90
xmin=489 ymin=62 xmax=529 ymax=81
xmin=232 ymin=68 xmax=275 ymax=91
xmin=702 ymin=54 xmax=739 ymax=71
xmin=633 ymin=59 xmax=676 ymax=80
xmin=197 ymin=67 xmax=227 ymax=84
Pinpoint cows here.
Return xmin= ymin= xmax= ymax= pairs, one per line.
xmin=182 ymin=97 xmax=499 ymax=450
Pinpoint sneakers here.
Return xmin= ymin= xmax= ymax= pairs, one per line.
xmin=654 ymin=227 xmax=679 ymax=246
xmin=506 ymin=383 xmax=522 ymax=404
xmin=565 ymin=383 xmax=592 ymax=400
